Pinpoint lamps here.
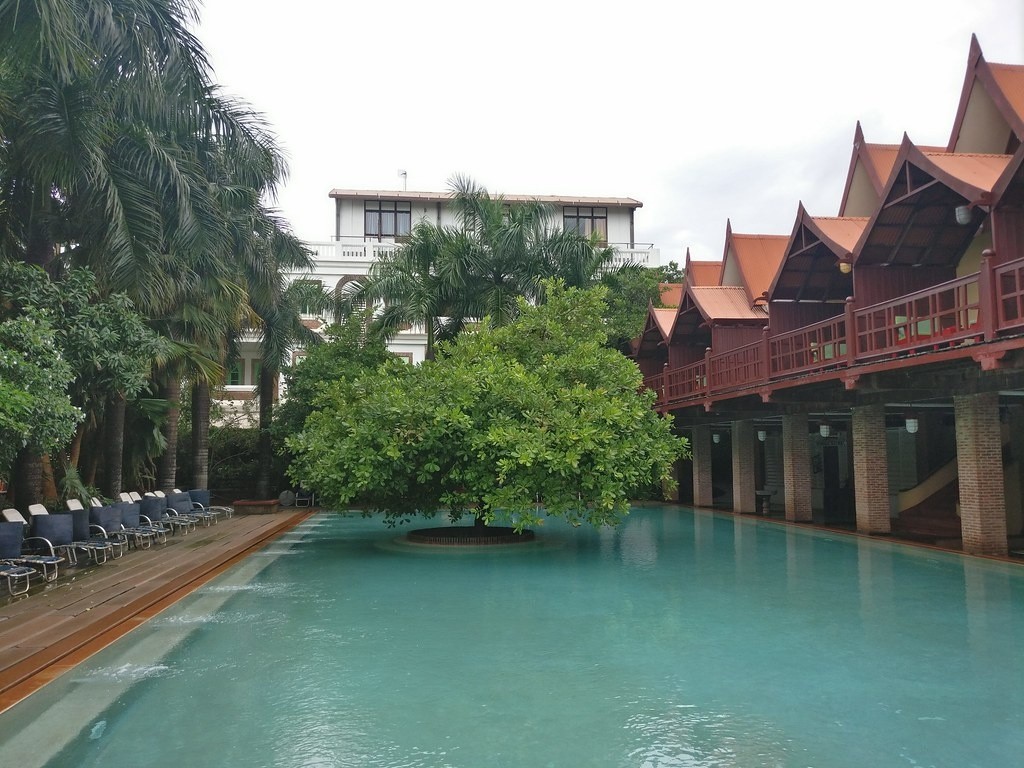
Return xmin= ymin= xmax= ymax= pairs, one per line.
xmin=839 ymin=262 xmax=852 ymax=274
xmin=713 ymin=420 xmax=721 ymax=443
xmin=955 ymin=206 xmax=979 ymax=226
xmin=818 ymin=394 xmax=831 ymax=438
xmin=758 ymin=417 xmax=767 ymax=441
xmin=905 ymin=402 xmax=919 ymax=434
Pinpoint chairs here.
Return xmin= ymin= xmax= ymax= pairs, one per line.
xmin=295 ymin=492 xmax=311 ymax=508
xmin=0 ymin=489 xmax=235 ymax=596
xmin=894 ymin=315 xmax=941 ymax=345
xmin=809 ymin=343 xmax=847 ymax=363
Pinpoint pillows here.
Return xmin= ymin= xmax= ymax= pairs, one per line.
xmin=167 ymin=493 xmax=191 ymax=516
xmin=143 ymin=495 xmax=169 ymax=521
xmin=0 ymin=521 xmax=23 ymax=559
xmin=62 ymin=509 xmax=90 ymax=542
xmin=112 ymin=502 xmax=140 ymax=528
xmin=189 ymin=490 xmax=210 ymax=508
xmin=30 ymin=515 xmax=73 ymax=546
xmin=136 ymin=499 xmax=161 ymax=522
xmin=90 ymin=507 xmax=122 ymax=533
